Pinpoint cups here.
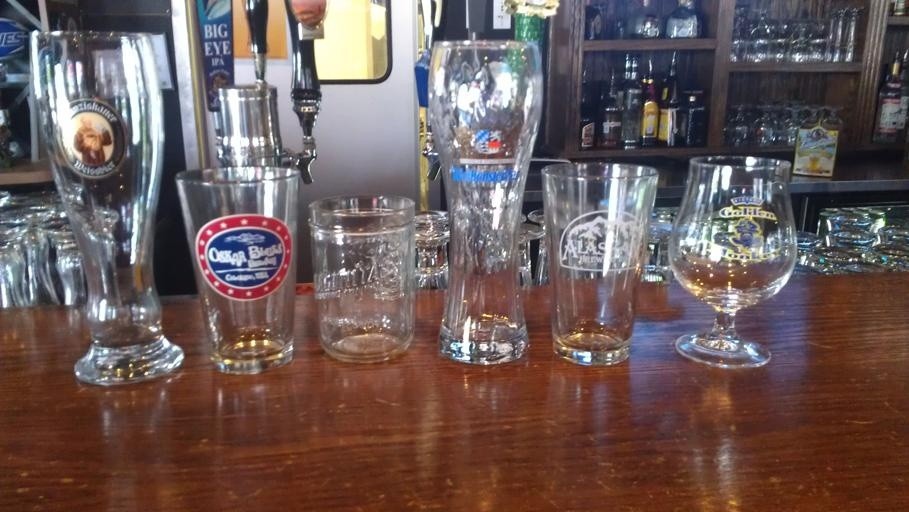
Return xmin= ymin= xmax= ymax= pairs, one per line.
xmin=175 ymin=166 xmax=301 ymax=374
xmin=429 ymin=40 xmax=545 ymax=365
xmin=541 ymin=163 xmax=659 ymax=367
xmin=839 ymin=6 xmax=861 ymax=61
xmin=824 ymin=8 xmax=841 ymax=62
xmin=28 ymin=29 xmax=184 ymax=388
xmin=775 ymin=20 xmax=830 ymax=62
xmin=622 ymin=103 xmax=642 ymax=150
xmin=308 ymin=193 xmax=416 ymax=364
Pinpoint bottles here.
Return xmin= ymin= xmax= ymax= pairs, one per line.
xmin=623 ymin=60 xmax=641 ymax=148
xmin=582 ymin=67 xmax=595 ymax=149
xmin=874 ymin=51 xmax=901 ymax=133
xmin=642 ymin=61 xmax=658 ymax=147
xmin=659 ymin=53 xmax=678 ymax=144
xmin=596 ymin=69 xmax=621 ymax=148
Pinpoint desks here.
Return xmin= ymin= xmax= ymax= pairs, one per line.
xmin=1 ymin=265 xmax=908 ymax=511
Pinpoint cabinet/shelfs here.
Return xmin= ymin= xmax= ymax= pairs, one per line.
xmin=546 ymin=0 xmax=908 ymax=158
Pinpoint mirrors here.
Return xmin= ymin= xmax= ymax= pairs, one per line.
xmin=318 ymin=0 xmax=392 ymax=85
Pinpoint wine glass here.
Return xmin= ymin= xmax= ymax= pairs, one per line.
xmin=413 ymin=215 xmax=452 ymax=290
xmin=798 ymin=210 xmax=909 ymax=273
xmin=802 ymin=104 xmax=824 ymax=128
xmin=821 ymin=104 xmax=845 ymax=130
xmin=0 ymin=188 xmax=86 ymax=306
xmin=669 ymin=155 xmax=798 ymax=369
xmin=520 ymin=208 xmax=549 ymax=287
xmin=784 ymin=100 xmax=805 ymax=125
xmin=727 ymin=5 xmax=748 ymax=63
xmin=650 ymin=207 xmax=681 ymax=267
xmin=754 ymin=105 xmax=781 ymax=146
xmin=747 ymin=8 xmax=774 ymax=63
xmin=781 ymin=107 xmax=805 ymax=145
xmin=725 ymin=103 xmax=749 ymax=144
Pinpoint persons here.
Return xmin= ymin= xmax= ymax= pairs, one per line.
xmin=72 ymin=115 xmax=114 ymax=169
